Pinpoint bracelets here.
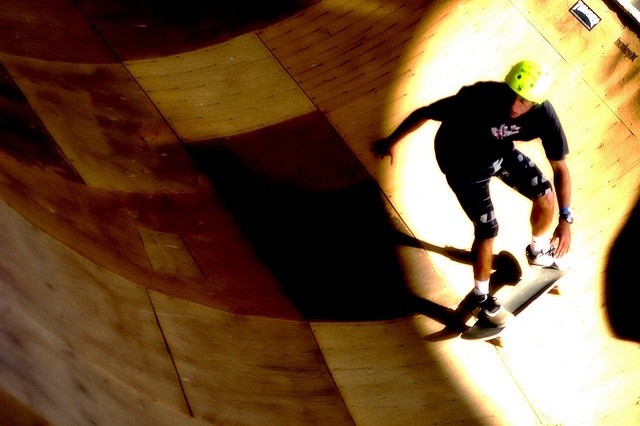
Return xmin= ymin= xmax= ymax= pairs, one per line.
xmin=559 ymin=208 xmax=571 ymax=213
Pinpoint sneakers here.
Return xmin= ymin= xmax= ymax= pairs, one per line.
xmin=467 ymin=294 xmax=514 ymax=328
xmin=526 ymin=243 xmax=570 ymax=271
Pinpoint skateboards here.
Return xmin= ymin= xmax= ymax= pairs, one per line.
xmin=460 ymin=264 xmax=565 ymax=347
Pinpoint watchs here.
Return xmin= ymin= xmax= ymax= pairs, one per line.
xmin=559 ymin=213 xmax=574 ymax=223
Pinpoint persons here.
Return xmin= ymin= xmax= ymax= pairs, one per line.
xmin=371 ymin=59 xmax=571 ymax=328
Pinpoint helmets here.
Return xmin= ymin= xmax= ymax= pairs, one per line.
xmin=505 ymin=59 xmax=554 ymax=104
